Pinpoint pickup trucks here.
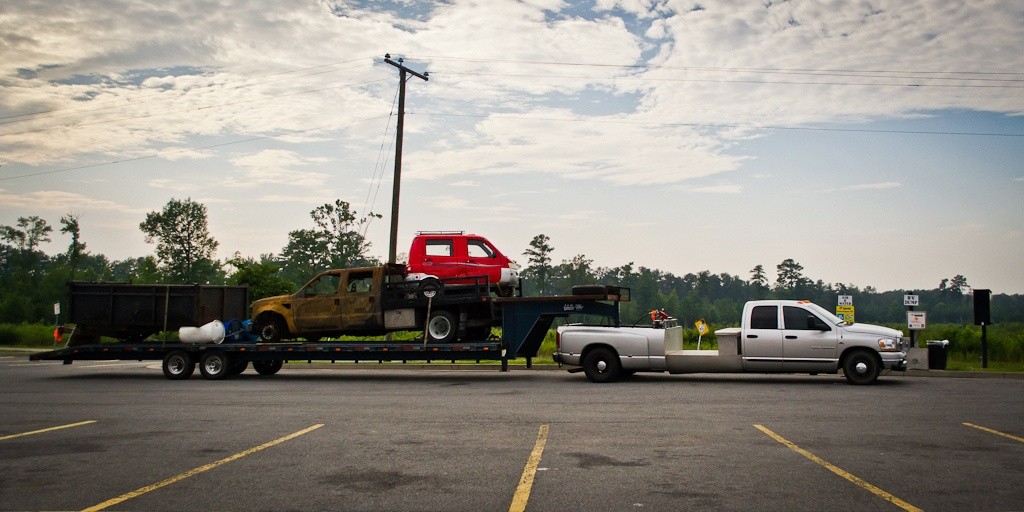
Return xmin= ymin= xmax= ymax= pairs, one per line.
xmin=553 ymin=298 xmax=908 ymax=385
xmin=248 ymin=263 xmax=516 ymax=343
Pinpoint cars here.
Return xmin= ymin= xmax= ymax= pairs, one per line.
xmin=407 ymin=232 xmax=520 ymax=296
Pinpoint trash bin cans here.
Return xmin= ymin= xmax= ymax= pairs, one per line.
xmin=926 ymin=338 xmax=949 ymax=369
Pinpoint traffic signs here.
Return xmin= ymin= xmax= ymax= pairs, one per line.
xmin=907 ymin=311 xmax=926 ymax=329
